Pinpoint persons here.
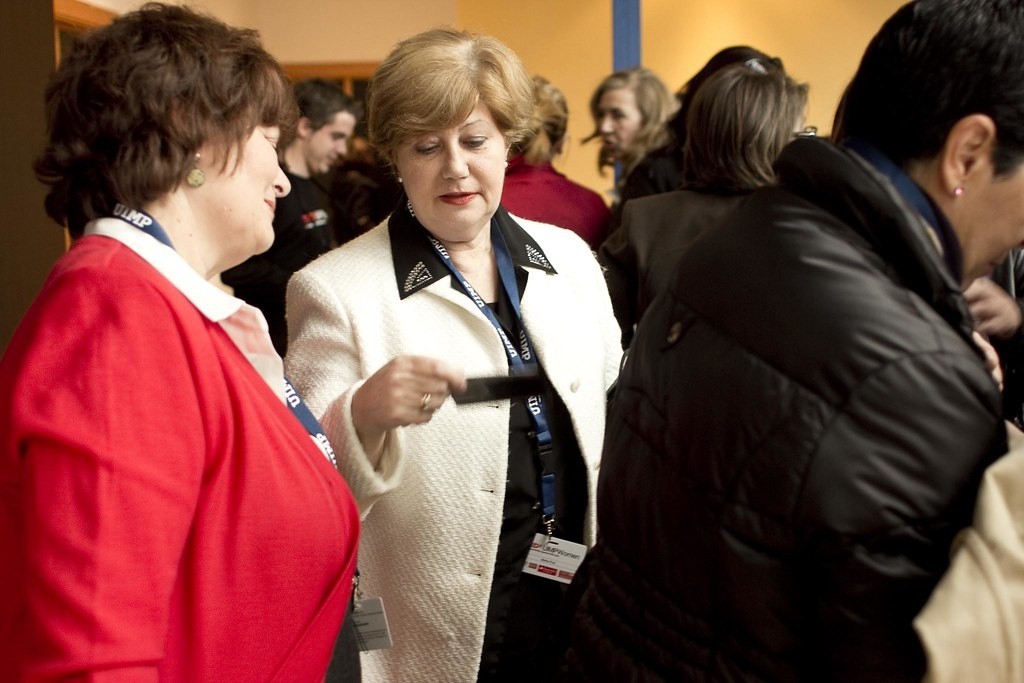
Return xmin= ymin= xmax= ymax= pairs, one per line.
xmin=0 ymin=0 xmax=1024 ymax=683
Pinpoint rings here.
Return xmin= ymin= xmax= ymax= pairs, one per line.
xmin=421 ymin=393 xmax=431 ymax=410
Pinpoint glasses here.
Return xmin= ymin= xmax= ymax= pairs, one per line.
xmin=787 ymin=126 xmax=819 ymax=138
